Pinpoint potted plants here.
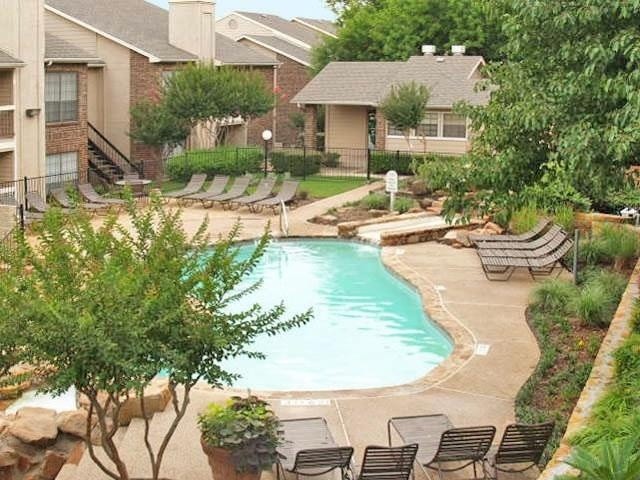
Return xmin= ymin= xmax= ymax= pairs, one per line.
xmin=197 ymin=391 xmax=291 ymax=480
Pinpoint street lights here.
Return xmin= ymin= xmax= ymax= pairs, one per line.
xmin=261 ymin=129 xmax=273 ymax=178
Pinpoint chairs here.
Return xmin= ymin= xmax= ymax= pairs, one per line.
xmin=160 ymin=173 xmax=299 ymax=214
xmin=267 ymin=413 xmax=555 ymax=480
xmin=24 ymin=172 xmax=152 ymax=230
xmin=469 ymin=217 xmax=573 ymax=285
